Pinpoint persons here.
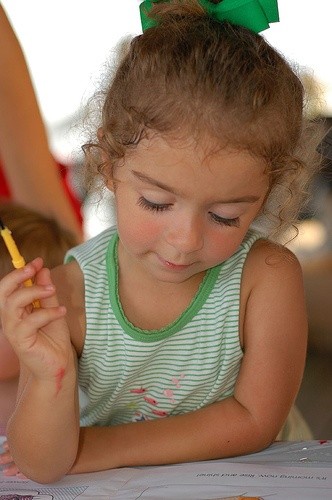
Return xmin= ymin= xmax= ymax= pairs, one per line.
xmin=0 ymin=0 xmax=332 ymax=439
xmin=0 ymin=1 xmax=322 ymax=484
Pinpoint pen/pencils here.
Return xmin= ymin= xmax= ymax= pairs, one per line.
xmin=0 ymin=219 xmax=41 ymax=309
